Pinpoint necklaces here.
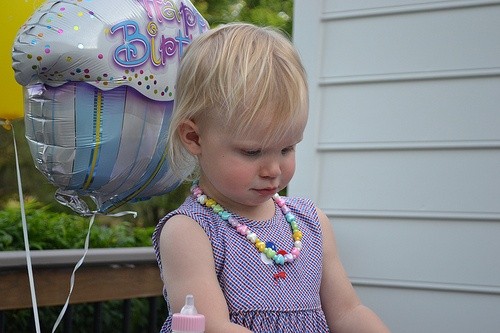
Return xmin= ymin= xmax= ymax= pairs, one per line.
xmin=189 ymin=184 xmax=302 ymax=265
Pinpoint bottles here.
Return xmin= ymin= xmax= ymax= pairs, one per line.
xmin=171 ymin=295 xmax=205 ymax=333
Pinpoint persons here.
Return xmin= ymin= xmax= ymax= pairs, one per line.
xmin=151 ymin=20 xmax=392 ymax=333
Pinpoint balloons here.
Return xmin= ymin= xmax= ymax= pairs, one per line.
xmin=0 ymin=0 xmax=54 ymax=129
xmin=13 ymin=1 xmax=211 ymax=218
